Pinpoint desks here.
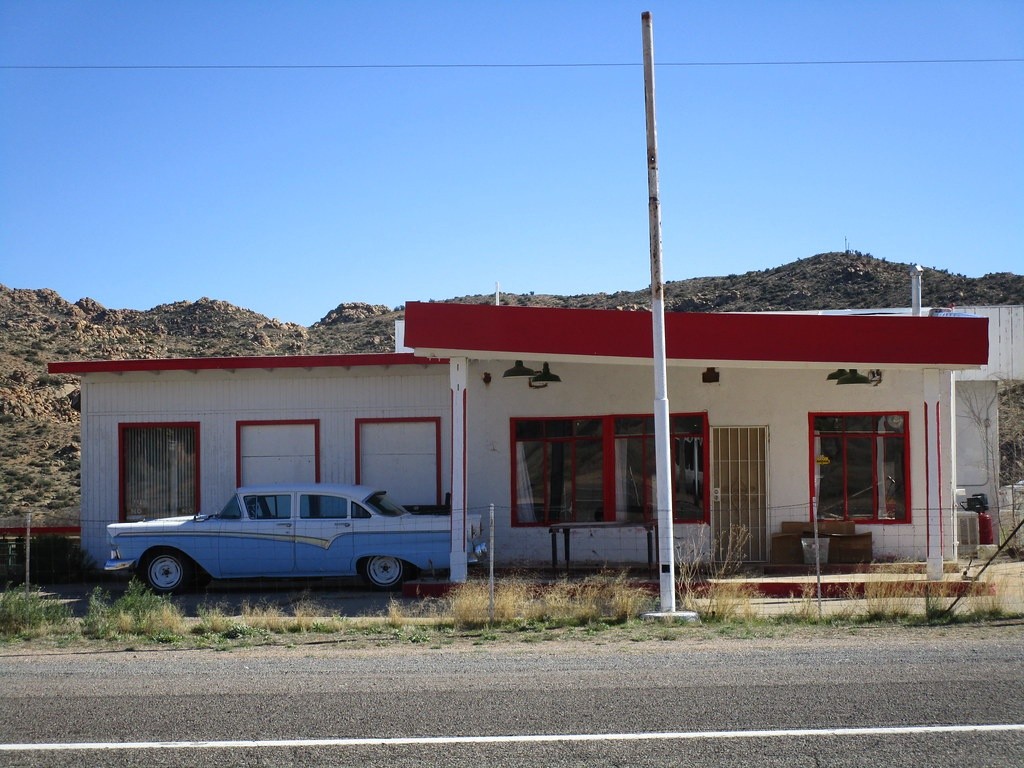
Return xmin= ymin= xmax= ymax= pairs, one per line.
xmin=551 ymin=522 xmax=660 ymax=582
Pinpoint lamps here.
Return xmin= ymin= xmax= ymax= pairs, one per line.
xmin=828 ymin=369 xmax=871 ymax=385
xmin=531 ymin=361 xmax=562 ymax=382
xmin=503 ymin=359 xmax=536 ymax=378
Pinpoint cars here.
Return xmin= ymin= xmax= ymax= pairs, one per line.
xmin=104 ymin=485 xmax=486 ymax=600
